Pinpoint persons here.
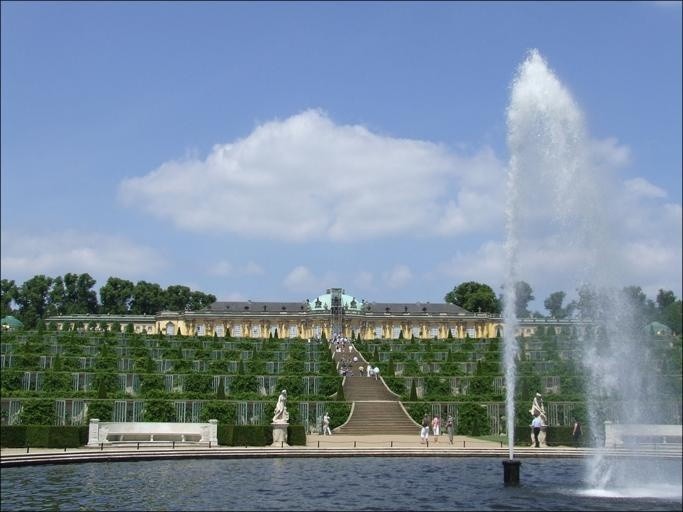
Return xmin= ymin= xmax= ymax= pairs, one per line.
xmin=431 ymin=413 xmax=441 ymax=444
xmin=419 ymin=413 xmax=430 ymax=444
xmin=321 ymin=411 xmax=332 ymax=436
xmin=443 ymin=414 xmax=455 ymax=445
xmin=528 ymin=411 xmax=542 ymax=448
xmin=531 ymin=391 xmax=548 ymax=424
xmin=330 ymin=330 xmax=380 ymax=381
xmin=570 ymin=416 xmax=584 ymax=447
xmin=270 ymin=389 xmax=288 ymax=422
